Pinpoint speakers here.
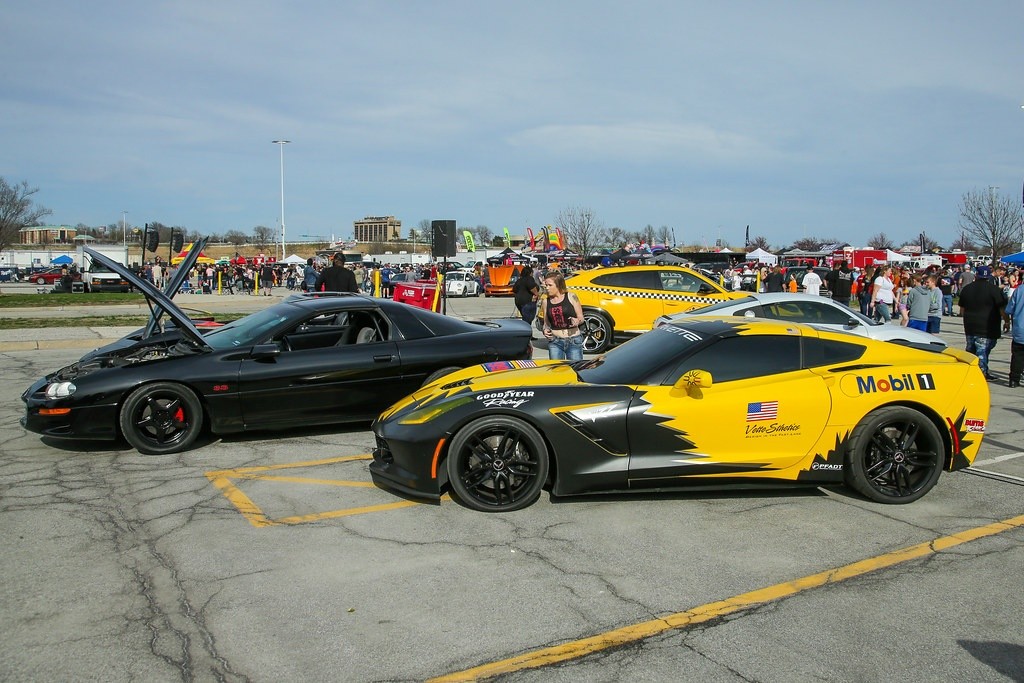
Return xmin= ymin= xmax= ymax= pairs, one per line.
xmin=147 ymin=231 xmax=159 ymax=252
xmin=431 ymin=220 xmax=456 ymax=258
xmin=174 ymin=234 xmax=183 ymax=253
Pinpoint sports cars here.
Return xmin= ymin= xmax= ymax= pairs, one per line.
xmin=368 ymin=320 xmax=990 ymax=511
xmin=485 ymin=265 xmax=529 ymax=297
xmin=14 ymin=233 xmax=537 ymax=455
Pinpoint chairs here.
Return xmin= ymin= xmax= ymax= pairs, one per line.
xmin=356 ymin=327 xmax=376 ymax=345
xmin=339 ymin=325 xmax=358 ymax=346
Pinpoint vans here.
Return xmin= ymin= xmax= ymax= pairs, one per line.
xmin=977 ymin=256 xmax=993 ymax=265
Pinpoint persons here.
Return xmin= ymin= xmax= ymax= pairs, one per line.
xmin=907 ymin=274 xmax=932 ymax=332
xmin=1005 ymin=274 xmax=1024 ymax=388
xmin=512 ymin=266 xmax=540 ymax=346
xmin=542 ymin=272 xmax=584 ymax=359
xmin=315 ymin=253 xmax=359 ymax=297
xmin=958 ymin=266 xmax=1010 ymax=381
xmin=62 ymin=257 xmax=1024 ymax=326
xmin=925 ymin=275 xmax=943 ymax=337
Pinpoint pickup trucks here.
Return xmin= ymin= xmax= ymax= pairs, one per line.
xmin=457 ymin=260 xmax=485 ymax=273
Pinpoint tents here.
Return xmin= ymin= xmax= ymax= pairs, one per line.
xmin=50 ymin=255 xmax=73 ymax=263
xmin=278 ymin=254 xmax=307 ymax=264
xmin=648 ymin=252 xmax=688 ymax=266
xmin=172 ymin=242 xmax=215 ymax=264
xmin=746 ymin=248 xmax=778 ymax=267
xmin=1000 ymin=251 xmax=1024 ymax=266
xmin=719 ymin=247 xmax=734 ymax=253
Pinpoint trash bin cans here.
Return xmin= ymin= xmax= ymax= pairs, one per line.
xmin=393 ymin=280 xmax=444 ymax=314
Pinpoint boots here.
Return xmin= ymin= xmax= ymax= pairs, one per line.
xmin=891 ymin=312 xmax=899 ymax=319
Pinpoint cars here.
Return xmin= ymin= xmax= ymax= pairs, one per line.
xmin=784 ymin=265 xmax=833 ymax=299
xmin=345 ymin=261 xmax=398 ymax=287
xmin=436 ymin=261 xmax=464 ymax=272
xmin=443 ymin=272 xmax=481 ymax=297
xmin=538 ymin=265 xmax=803 ymax=355
xmin=29 ymin=267 xmax=71 ymax=285
xmin=649 ymin=292 xmax=950 ymax=349
xmin=388 ymin=273 xmax=408 ymax=296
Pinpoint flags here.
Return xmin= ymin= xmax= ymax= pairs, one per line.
xmin=527 ymin=227 xmax=534 ymax=251
xmin=540 ymin=226 xmax=549 ymax=252
xmin=503 ymin=226 xmax=510 ymax=248
xmin=555 ymin=227 xmax=562 ymax=249
xmin=462 ymin=230 xmax=475 ymax=253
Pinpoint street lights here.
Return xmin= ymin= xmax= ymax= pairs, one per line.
xmin=123 ymin=211 xmax=128 ymax=247
xmin=272 ymin=139 xmax=291 ymax=261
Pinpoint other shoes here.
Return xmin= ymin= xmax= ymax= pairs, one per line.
xmin=985 ymin=373 xmax=998 ymax=382
xmin=531 ymin=337 xmax=538 ymax=340
xmin=264 ymin=291 xmax=266 ymax=296
xmin=1009 ymin=379 xmax=1020 ymax=387
xmin=268 ymin=294 xmax=271 ymax=296
xmin=1021 ymin=370 xmax=1024 ymax=380
xmin=958 ymin=313 xmax=962 ymax=317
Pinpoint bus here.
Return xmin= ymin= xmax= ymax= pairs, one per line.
xmin=399 ymin=263 xmax=414 ymax=272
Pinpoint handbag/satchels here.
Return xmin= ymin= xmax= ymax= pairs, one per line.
xmin=301 ymin=281 xmax=307 ymax=290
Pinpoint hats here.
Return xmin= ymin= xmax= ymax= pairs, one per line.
xmin=976 ymin=266 xmax=992 ymax=278
xmin=964 ymin=264 xmax=970 ymax=269
xmin=743 ymin=265 xmax=748 ymax=269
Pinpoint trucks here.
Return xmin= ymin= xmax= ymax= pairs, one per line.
xmin=75 ymin=244 xmax=132 ymax=292
xmin=903 ymin=255 xmax=942 ymax=273
